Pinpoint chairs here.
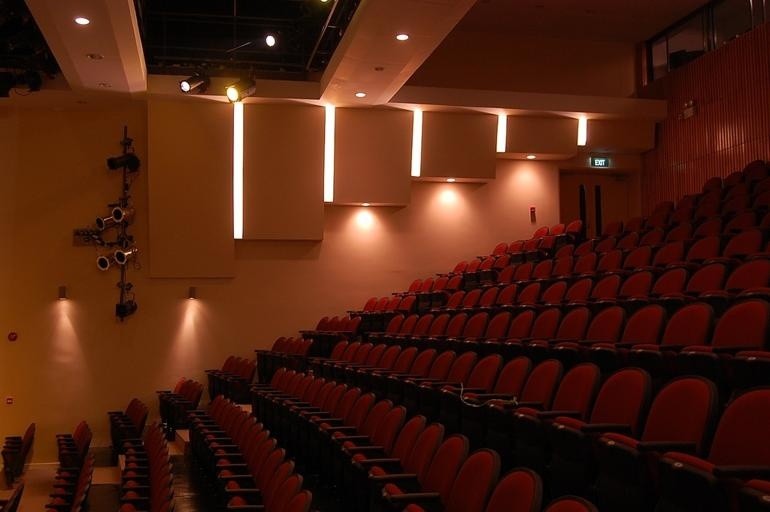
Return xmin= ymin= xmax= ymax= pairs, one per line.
xmin=0 ymin=173 xmax=770 ymax=512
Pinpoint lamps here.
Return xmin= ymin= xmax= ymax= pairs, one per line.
xmin=94 ymin=125 xmax=140 ymax=323
xmin=179 ymin=71 xmax=256 ymax=102
